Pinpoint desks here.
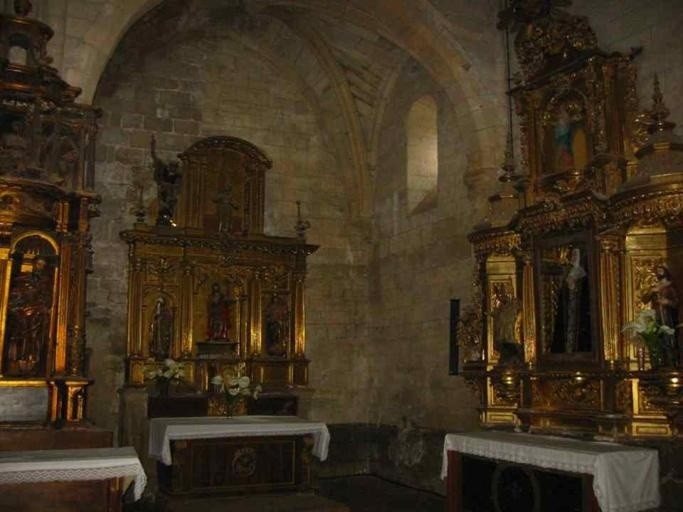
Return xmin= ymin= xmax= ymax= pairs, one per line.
xmin=438 ymin=427 xmax=663 ymax=512
xmin=145 ymin=412 xmax=330 ymax=498
xmin=0 ymin=444 xmax=148 ymax=509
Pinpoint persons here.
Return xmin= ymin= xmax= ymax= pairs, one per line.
xmin=149 ymin=298 xmax=173 ymax=361
xmin=150 ymin=139 xmax=180 ymax=225
xmin=642 ymin=267 xmax=681 ymax=366
xmin=555 ymin=109 xmax=584 ymax=183
xmin=214 ymin=184 xmax=240 ymax=232
xmin=5 ymin=257 xmax=51 ymax=377
xmin=266 ymin=296 xmax=288 ymax=356
xmin=548 ymin=248 xmax=589 ymax=353
xmin=492 ymin=286 xmax=522 ymax=365
xmin=207 ymin=284 xmax=230 ymax=340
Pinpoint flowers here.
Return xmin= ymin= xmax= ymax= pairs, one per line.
xmin=614 ymin=304 xmax=676 ymax=369
xmin=204 ymin=365 xmax=262 ymax=418
xmin=137 ymin=355 xmax=195 ymax=390
